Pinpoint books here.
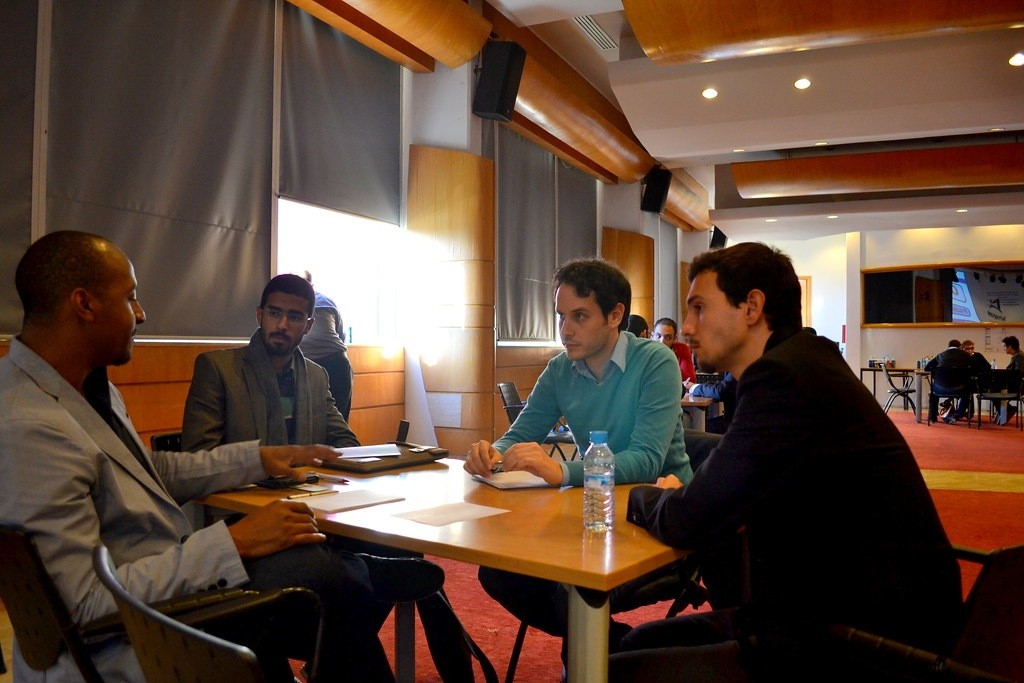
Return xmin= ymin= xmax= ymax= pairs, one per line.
xmin=473 ymin=470 xmax=547 ymax=489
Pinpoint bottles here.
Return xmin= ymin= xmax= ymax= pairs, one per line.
xmin=883 ymin=356 xmax=897 ymax=369
xmin=917 ymin=355 xmax=935 ymax=370
xmin=991 ymin=359 xmax=999 ymax=369
xmin=581 ymin=430 xmax=618 ymax=532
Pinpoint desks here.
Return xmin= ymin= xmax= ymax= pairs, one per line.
xmin=860 ymin=368 xmax=923 ymax=399
xmin=187 ymin=445 xmax=688 ymax=683
xmin=680 ymin=393 xmax=713 ymax=432
xmin=915 ymin=369 xmax=1007 ymax=422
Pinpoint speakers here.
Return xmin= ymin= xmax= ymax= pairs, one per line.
xmin=710 ymin=226 xmax=726 ymax=250
xmin=641 ymin=168 xmax=672 ymax=215
xmin=471 ymin=40 xmax=527 ymax=123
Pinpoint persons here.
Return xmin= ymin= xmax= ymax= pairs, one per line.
xmin=924 ymin=339 xmax=991 ymax=424
xmin=607 ymin=242 xmax=962 ymax=683
xmin=299 ymin=270 xmax=353 ymax=423
xmin=682 ymin=371 xmax=739 ymax=434
xmin=0 ymin=231 xmax=445 ymax=683
xmin=651 ymin=318 xmax=696 ymax=429
xmin=181 ymin=275 xmax=424 ymax=683
xmin=464 ymin=258 xmax=694 ymax=683
xmin=988 ymin=336 xmax=1024 ymax=425
xmin=627 ymin=315 xmax=686 ymax=399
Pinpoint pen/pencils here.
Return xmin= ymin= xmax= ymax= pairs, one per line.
xmin=308 ymin=471 xmax=349 ymax=483
xmin=493 ymin=461 xmax=504 ymax=465
xmin=287 ymin=491 xmax=339 ymax=498
xmin=685 ymin=377 xmax=690 ymax=385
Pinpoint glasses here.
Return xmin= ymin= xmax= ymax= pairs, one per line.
xmin=263 ymin=307 xmax=311 ymax=324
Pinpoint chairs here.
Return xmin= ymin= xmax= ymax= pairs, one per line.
xmin=927 ymin=366 xmax=1024 ymax=429
xmin=89 ymin=545 xmax=262 ymax=683
xmin=735 ymin=546 xmax=1024 ymax=683
xmin=0 ymin=522 xmax=325 ymax=683
xmin=504 ymin=431 xmax=727 ymax=683
xmin=147 ymin=434 xmax=423 ymax=683
xmin=497 ymin=381 xmax=581 ymax=461
xmin=881 ymin=364 xmax=916 ymax=413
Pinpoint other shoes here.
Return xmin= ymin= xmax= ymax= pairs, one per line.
xmin=926 ymin=415 xmax=937 ymax=422
xmin=943 ymin=416 xmax=958 ymax=424
